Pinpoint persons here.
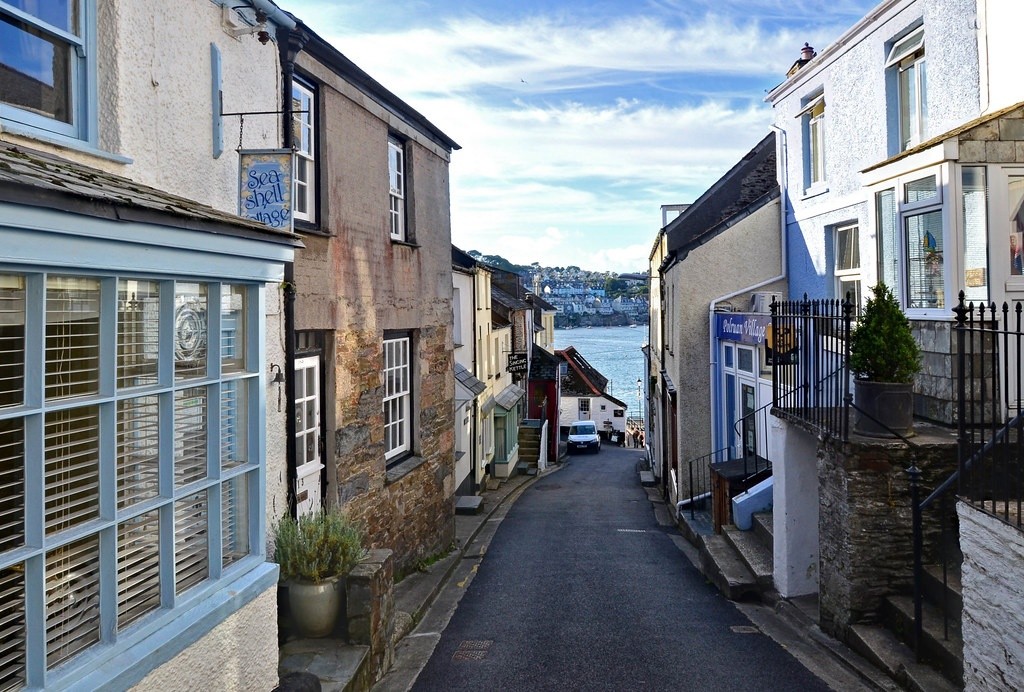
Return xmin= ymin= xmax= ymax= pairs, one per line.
xmin=639 ymin=428 xmax=646 ymax=449
xmin=633 ymin=427 xmax=640 ymax=449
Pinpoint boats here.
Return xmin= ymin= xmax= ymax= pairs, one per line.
xmin=587 ymin=326 xmax=593 ymax=329
xmin=630 ymin=324 xmax=636 ymax=327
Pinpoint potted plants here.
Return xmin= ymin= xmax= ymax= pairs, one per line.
xmin=267 ymin=479 xmax=369 ymax=640
xmin=846 ymin=281 xmax=925 ymax=438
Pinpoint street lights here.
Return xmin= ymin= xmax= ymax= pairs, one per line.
xmin=637 ymin=378 xmax=642 ymax=428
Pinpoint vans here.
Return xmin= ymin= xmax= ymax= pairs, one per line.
xmin=567 ymin=419 xmax=601 ymax=455
